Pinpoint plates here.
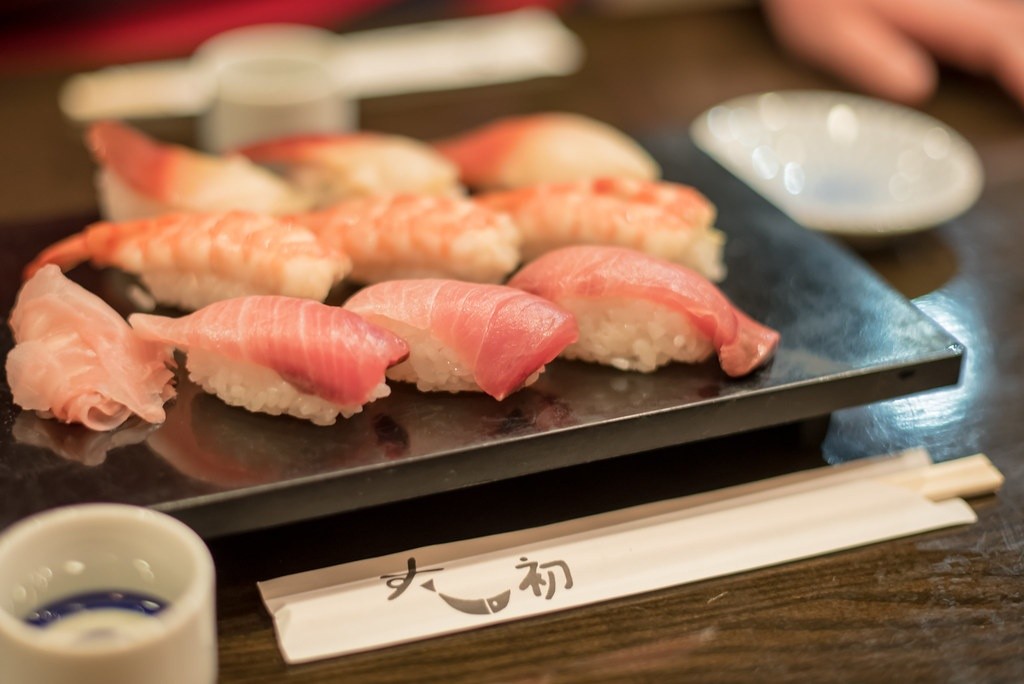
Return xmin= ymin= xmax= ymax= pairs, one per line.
xmin=691 ymin=89 xmax=985 ymax=236
xmin=0 ymin=130 xmax=967 ymax=538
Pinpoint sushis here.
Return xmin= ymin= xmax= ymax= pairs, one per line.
xmin=5 ymin=110 xmax=783 ymax=429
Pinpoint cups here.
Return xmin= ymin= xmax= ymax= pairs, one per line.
xmin=191 ymin=25 xmax=361 ymax=151
xmin=0 ymin=502 xmax=219 ymax=684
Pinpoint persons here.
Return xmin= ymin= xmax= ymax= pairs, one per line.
xmin=0 ymin=0 xmax=1024 ymax=102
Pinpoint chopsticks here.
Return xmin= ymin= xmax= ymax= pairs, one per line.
xmin=909 ymin=451 xmax=1005 ymax=504
